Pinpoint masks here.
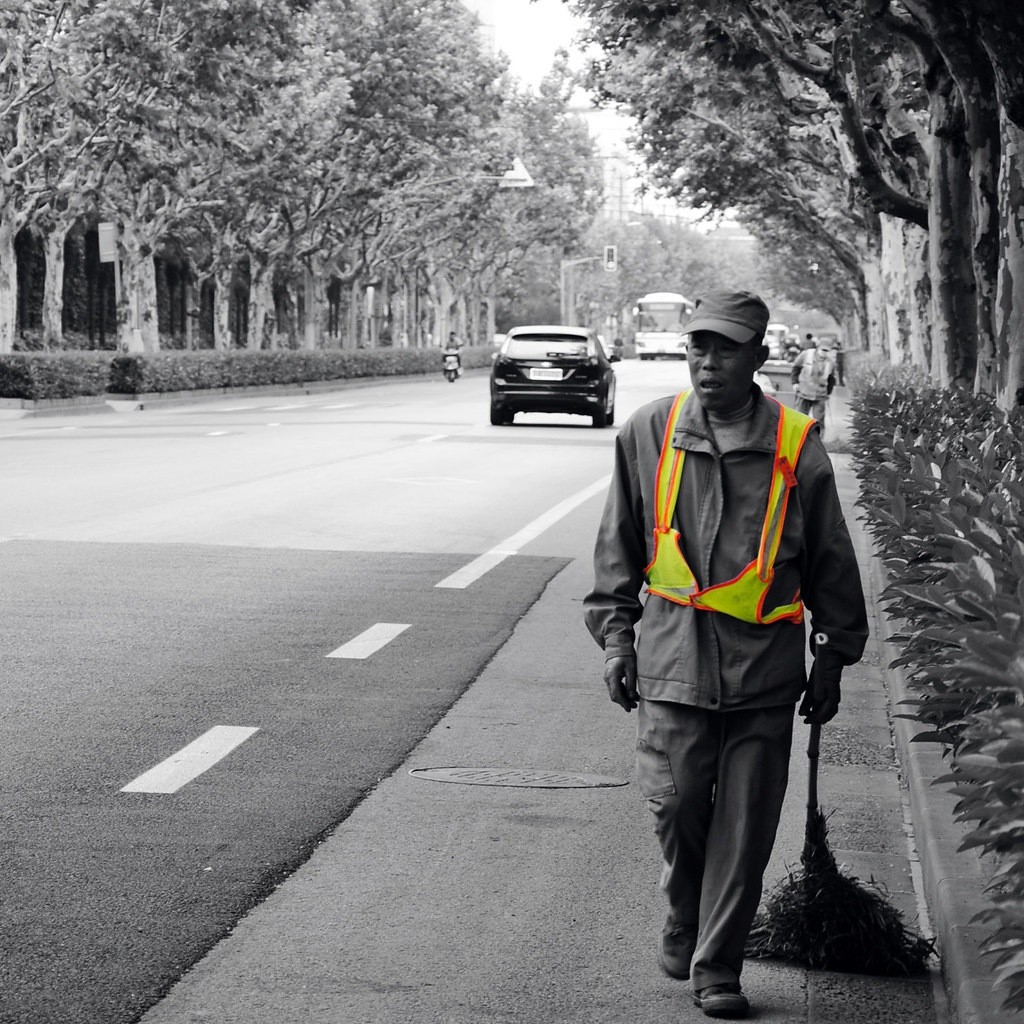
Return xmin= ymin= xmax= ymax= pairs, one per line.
xmin=818 ymin=349 xmax=831 ymax=359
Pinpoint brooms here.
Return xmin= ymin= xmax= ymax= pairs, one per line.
xmin=744 ymin=631 xmax=943 ymax=981
xmin=822 ymin=399 xmax=856 ymax=454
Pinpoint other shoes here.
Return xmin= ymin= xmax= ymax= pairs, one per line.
xmin=840 ymin=382 xmax=845 ymax=387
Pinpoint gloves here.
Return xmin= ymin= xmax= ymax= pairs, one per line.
xmin=793 ymin=384 xmax=800 ymax=392
xmin=826 ymin=394 xmax=830 ymax=400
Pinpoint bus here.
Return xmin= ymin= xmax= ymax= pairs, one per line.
xmin=632 ymin=292 xmax=695 ymax=361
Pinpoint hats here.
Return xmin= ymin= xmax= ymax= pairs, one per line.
xmin=819 ymin=336 xmax=832 ymax=350
xmin=680 ymin=289 xmax=770 ymax=347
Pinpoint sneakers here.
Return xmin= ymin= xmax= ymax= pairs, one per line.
xmin=659 ymin=928 xmax=693 ymax=980
xmin=694 ymin=983 xmax=750 ymax=1019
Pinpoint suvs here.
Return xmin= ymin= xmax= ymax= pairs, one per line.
xmin=489 ymin=325 xmax=621 ymax=429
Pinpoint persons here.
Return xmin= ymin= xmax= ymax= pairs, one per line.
xmin=444 ymin=331 xmax=461 ymax=350
xmin=585 ymin=289 xmax=869 ymax=1016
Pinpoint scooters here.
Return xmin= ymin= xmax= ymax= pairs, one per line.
xmin=437 ymin=342 xmax=465 ymax=384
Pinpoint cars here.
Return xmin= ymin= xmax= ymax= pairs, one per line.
xmin=759 ymin=322 xmax=802 ymax=362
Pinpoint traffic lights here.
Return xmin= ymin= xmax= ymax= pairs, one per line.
xmin=604 ymin=245 xmax=619 ymax=274
